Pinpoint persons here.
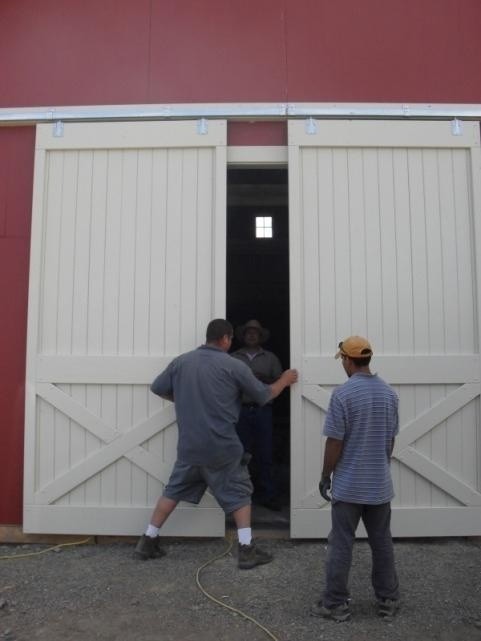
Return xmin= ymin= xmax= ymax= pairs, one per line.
xmin=309 ymin=336 xmax=403 ymax=622
xmin=134 ymin=317 xmax=299 ymax=570
xmin=229 ymin=318 xmax=283 ymax=510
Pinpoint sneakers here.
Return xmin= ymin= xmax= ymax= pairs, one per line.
xmin=310 ymin=598 xmax=351 ymax=622
xmin=133 ymin=534 xmax=167 ymax=560
xmin=238 ymin=538 xmax=273 ymax=569
xmin=378 ymin=594 xmax=405 ymax=617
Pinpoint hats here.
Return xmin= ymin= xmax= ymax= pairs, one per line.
xmin=335 ymin=336 xmax=373 ymax=359
xmin=235 ymin=320 xmax=270 ymax=343
